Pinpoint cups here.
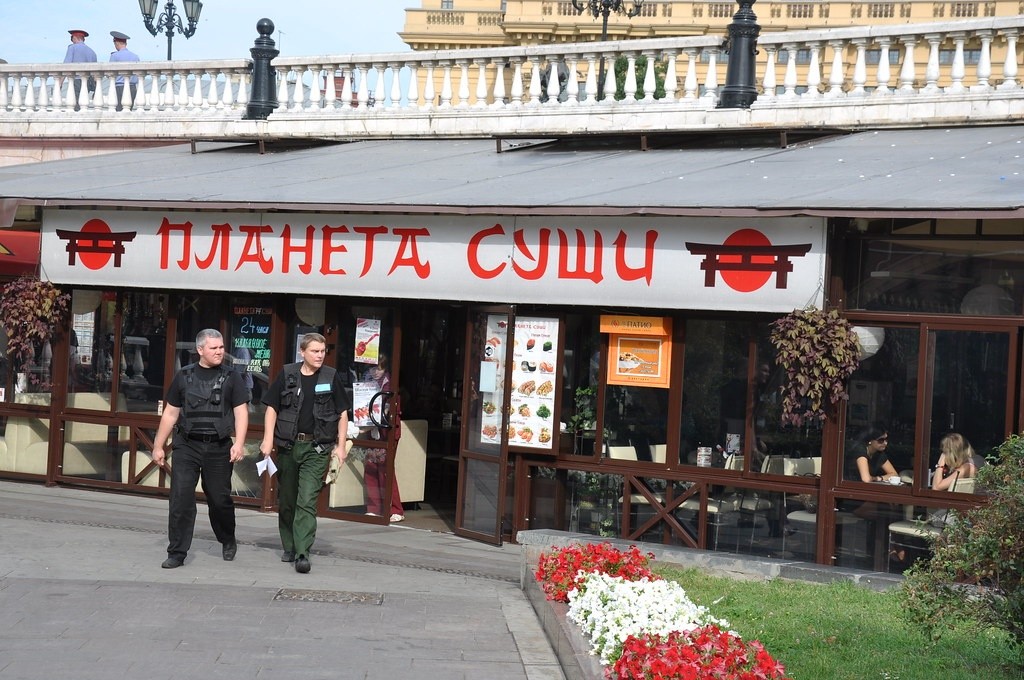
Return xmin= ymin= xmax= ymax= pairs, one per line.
xmin=888 ymin=476 xmax=901 ymax=485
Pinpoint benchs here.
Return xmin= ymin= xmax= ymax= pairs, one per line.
xmin=0 ymin=391 xmax=429 ymax=513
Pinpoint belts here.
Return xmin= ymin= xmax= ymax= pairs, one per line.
xmin=188 ymin=433 xmax=224 ymax=443
xmin=295 ymin=432 xmax=313 ymax=442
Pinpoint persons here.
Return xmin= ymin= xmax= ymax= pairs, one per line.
xmin=58 ymin=29 xmax=97 ymax=112
xmin=837 ymin=425 xmax=907 ymax=562
xmin=359 ymin=353 xmax=405 ymax=522
xmin=152 ymin=330 xmax=250 ymax=569
xmin=932 ymin=433 xmax=989 ymax=525
xmin=260 ymin=333 xmax=352 ymax=574
xmin=105 ymin=31 xmax=140 ymax=111
xmin=231 ymin=334 xmax=253 ymax=405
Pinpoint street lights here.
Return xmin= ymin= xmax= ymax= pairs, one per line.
xmin=137 ymin=0 xmax=205 ymax=62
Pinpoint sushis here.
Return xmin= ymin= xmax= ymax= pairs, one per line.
xmin=486 ymin=337 xmax=554 ymax=373
xmin=518 ymin=381 xmax=553 ymax=396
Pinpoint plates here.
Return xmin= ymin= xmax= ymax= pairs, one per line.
xmin=618 ymin=352 xmax=640 ymax=368
xmin=483 ymin=337 xmax=556 ymax=447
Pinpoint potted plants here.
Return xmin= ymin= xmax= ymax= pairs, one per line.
xmin=0 ymin=270 xmax=72 ymax=388
xmin=566 ymin=385 xmax=608 ymax=456
xmin=568 ymin=470 xmax=623 ymax=539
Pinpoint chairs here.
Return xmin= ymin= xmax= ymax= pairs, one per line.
xmin=887 ymin=471 xmax=978 ymax=573
xmin=607 ymin=444 xmax=870 ymax=570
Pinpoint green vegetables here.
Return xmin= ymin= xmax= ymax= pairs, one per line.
xmin=536 ymin=405 xmax=551 ymax=419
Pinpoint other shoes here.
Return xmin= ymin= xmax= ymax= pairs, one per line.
xmin=281 ymin=550 xmax=296 ymax=562
xmin=296 ymin=557 xmax=310 ymax=572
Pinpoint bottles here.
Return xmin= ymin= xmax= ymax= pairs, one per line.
xmin=715 ymin=448 xmax=725 ymax=467
xmin=414 ymin=304 xmax=465 ymax=431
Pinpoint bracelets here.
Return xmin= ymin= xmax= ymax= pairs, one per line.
xmin=879 ymin=476 xmax=883 ymax=482
xmin=935 ymin=464 xmax=944 ymax=468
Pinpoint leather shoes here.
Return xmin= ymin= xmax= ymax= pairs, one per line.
xmin=223 ymin=538 xmax=236 ymax=560
xmin=162 ymin=557 xmax=183 ymax=567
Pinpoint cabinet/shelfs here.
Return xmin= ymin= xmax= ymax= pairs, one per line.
xmin=568 ymin=390 xmax=630 ymax=534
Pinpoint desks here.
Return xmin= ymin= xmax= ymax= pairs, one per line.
xmin=427 ymin=426 xmax=460 ymax=504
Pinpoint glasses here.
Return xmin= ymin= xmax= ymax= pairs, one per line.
xmin=872 ymin=436 xmax=888 ymax=444
